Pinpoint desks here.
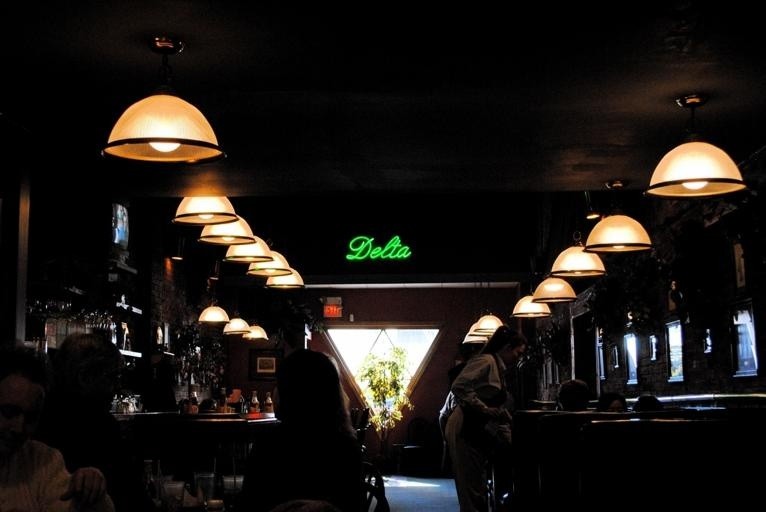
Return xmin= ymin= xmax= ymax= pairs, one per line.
xmin=192 ymin=416 xmax=272 ymax=493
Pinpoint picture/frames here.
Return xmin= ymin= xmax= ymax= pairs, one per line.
xmin=623 ymin=334 xmax=639 ymax=385
xmin=666 ymin=321 xmax=685 ymax=383
xmin=729 ymin=300 xmax=760 ymax=377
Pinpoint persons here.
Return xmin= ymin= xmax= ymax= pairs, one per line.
xmin=445 ymin=327 xmax=530 ymax=512
xmin=556 ymin=379 xmax=589 ymax=411
xmin=231 ymin=333 xmax=391 ymax=512
xmin=34 ymin=334 xmax=159 ymax=512
xmin=438 ymin=390 xmax=457 ymax=445
xmin=598 ymin=392 xmax=627 ymax=412
xmin=0 ymin=349 xmax=116 ymax=512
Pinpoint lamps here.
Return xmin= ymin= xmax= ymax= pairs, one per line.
xmin=101 ymin=36 xmax=224 ymax=162
xmin=173 ymin=197 xmax=238 ymax=225
xmin=197 ymin=214 xmax=255 ymax=247
xmin=549 ymin=231 xmax=607 ymax=278
xmin=511 ymin=295 xmax=552 ymax=318
xmin=247 ymin=251 xmax=293 ymax=277
xmin=532 ymin=275 xmax=577 ymax=306
xmin=243 ymin=320 xmax=268 ymax=342
xmin=265 ymin=268 xmax=305 ymax=290
xmin=462 ymin=282 xmax=505 ymax=342
xmin=224 ymin=237 xmax=271 ymax=262
xmin=585 ymin=179 xmax=652 ymax=252
xmin=224 ymin=309 xmax=249 ymax=336
xmin=198 ymin=288 xmax=229 ymax=324
xmin=643 ymin=95 xmax=747 ymax=199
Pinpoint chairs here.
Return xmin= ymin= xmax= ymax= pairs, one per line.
xmin=352 ymin=411 xmax=371 ymax=451
xmin=403 ymin=418 xmax=430 ymax=477
xmin=363 ymin=462 xmax=386 ymax=493
xmin=363 ymin=483 xmax=391 ymax=512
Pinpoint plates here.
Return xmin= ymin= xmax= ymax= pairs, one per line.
xmin=191 ymin=412 xmax=238 ymax=417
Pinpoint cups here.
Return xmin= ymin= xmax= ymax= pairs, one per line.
xmin=193 ymin=471 xmax=215 ymax=506
xmin=222 ymin=473 xmax=245 ymax=496
xmin=145 ymin=460 xmax=159 ymax=502
xmin=162 ymin=483 xmax=184 ymax=511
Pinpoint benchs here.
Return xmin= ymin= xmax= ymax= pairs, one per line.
xmin=487 ymin=411 xmax=739 ymax=512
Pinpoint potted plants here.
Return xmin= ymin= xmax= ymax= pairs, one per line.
xmin=355 ymin=346 xmax=415 ymax=474
xmin=169 ymin=318 xmax=227 ymax=414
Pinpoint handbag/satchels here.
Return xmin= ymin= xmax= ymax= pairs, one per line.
xmin=485 ymin=407 xmax=513 ymax=445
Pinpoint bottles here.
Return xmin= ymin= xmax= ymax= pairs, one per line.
xmin=217 ymin=386 xmax=226 ymax=408
xmin=250 ymin=391 xmax=259 ymax=411
xmin=264 ymin=392 xmax=273 ymax=411
xmin=23 ymin=299 xmax=134 ymax=351
